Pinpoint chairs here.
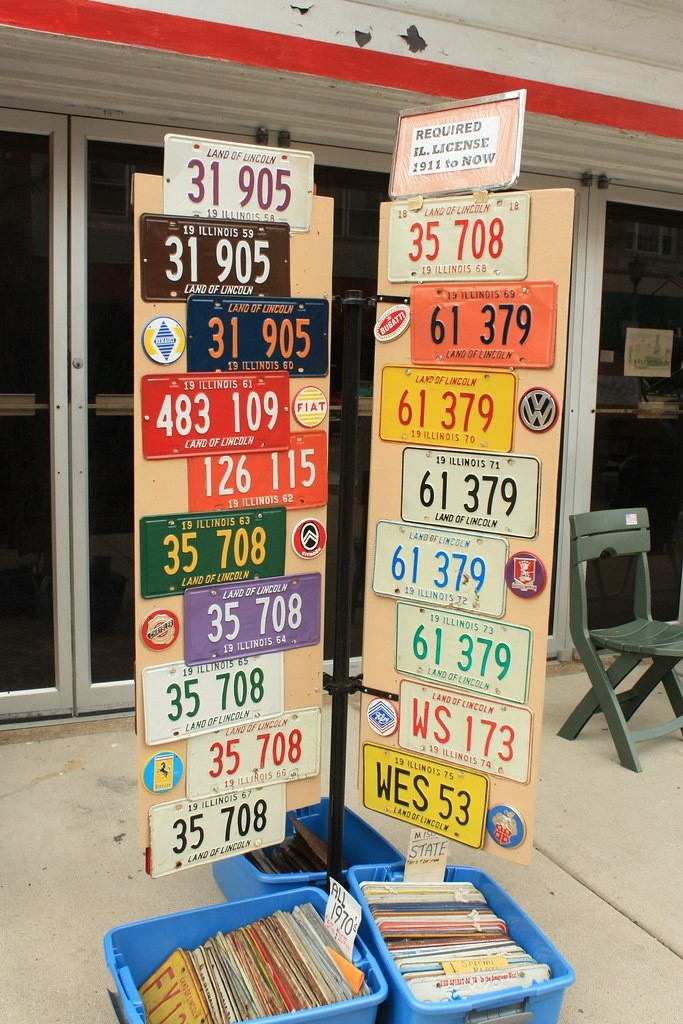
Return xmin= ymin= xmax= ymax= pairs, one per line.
xmin=555 ymin=508 xmax=683 ymax=773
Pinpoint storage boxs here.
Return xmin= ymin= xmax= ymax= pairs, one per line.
xmin=106 ymin=886 xmax=390 ymax=1024
xmin=348 ymin=863 xmax=576 ymax=1024
xmin=215 ymin=797 xmax=406 ymax=902
